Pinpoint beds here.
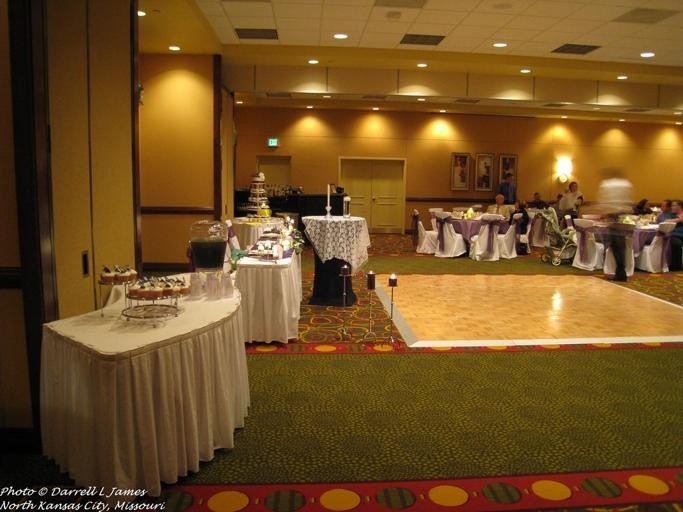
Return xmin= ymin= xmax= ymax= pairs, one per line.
xmin=41 ymin=216 xmax=302 ymax=500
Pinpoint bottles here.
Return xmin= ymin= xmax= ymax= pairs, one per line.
xmin=187 ymin=270 xmax=234 ymax=302
xmin=237 ymin=182 xmax=304 ymax=212
xmin=225 ymin=219 xmax=240 ymax=253
xmin=273 ymin=238 xmax=283 ymax=262
xmin=451 ymin=210 xmax=479 ymax=219
xmin=343 ymin=197 xmax=351 ymax=218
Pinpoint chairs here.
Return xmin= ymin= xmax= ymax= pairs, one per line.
xmin=564 ymin=214 xmax=683 ymax=280
xmin=410 ymin=205 xmax=549 ymax=261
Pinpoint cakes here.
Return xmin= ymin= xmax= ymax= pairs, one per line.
xmin=246 ymin=172 xmax=269 ymax=219
xmin=100 ymin=264 xmax=190 ymax=298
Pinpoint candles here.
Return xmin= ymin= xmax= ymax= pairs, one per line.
xmin=327 ymin=184 xmax=330 ymax=206
xmin=341 ymin=264 xmax=350 ymax=274
xmin=388 ymin=273 xmax=397 ymax=286
xmin=367 ymin=270 xmax=376 ymax=289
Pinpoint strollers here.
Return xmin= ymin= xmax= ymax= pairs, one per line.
xmin=537 ymin=208 xmax=577 ymax=266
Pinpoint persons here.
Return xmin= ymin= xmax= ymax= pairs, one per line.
xmin=485 ymin=193 xmax=510 ymax=223
xmin=496 ymin=172 xmax=517 ymax=205
xmin=632 ymin=199 xmax=653 ymax=214
xmin=507 ymin=198 xmax=529 ymax=234
xmin=655 ymin=199 xmax=677 ymax=225
xmin=557 ymin=181 xmax=584 ymax=218
xmin=583 ymin=165 xmax=633 ymax=282
xmin=664 ymin=199 xmax=682 ymax=271
xmin=527 ymin=191 xmax=551 ymax=210
xmin=549 ymin=194 xmax=563 ymax=204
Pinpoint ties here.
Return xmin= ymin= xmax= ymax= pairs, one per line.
xmin=496 ymin=206 xmax=500 ymax=214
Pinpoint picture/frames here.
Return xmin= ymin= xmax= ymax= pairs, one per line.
xmin=451 ymin=152 xmax=518 ymax=192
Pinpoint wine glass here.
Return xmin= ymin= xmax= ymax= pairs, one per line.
xmin=639 ymin=214 xmax=652 ymax=225
